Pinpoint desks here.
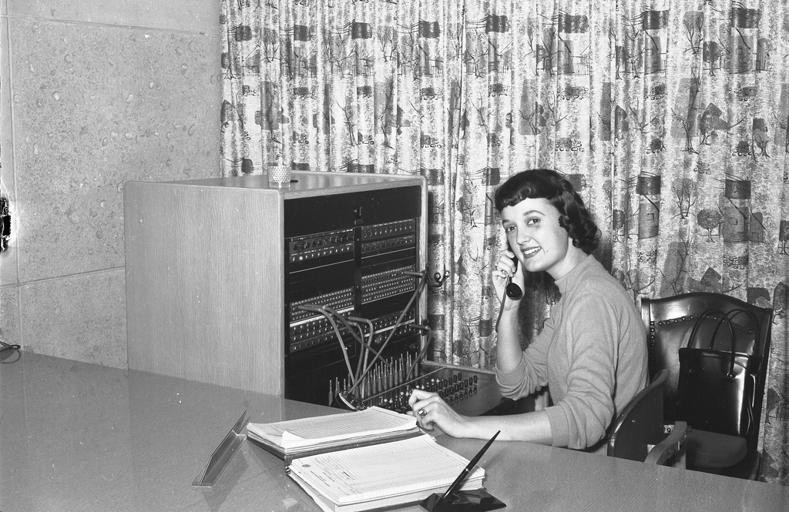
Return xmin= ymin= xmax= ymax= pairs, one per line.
xmin=1 ymin=347 xmax=787 ymax=511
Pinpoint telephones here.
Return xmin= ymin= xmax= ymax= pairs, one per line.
xmin=506 ymin=242 xmax=522 ymax=300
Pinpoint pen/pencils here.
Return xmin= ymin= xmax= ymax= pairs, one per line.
xmin=440 ymin=430 xmax=502 ymax=501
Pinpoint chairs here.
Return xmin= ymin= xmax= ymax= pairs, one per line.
xmin=607 ymin=292 xmax=776 ymax=480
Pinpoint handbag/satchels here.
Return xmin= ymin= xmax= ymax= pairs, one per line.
xmin=678 ymin=308 xmax=761 ymax=435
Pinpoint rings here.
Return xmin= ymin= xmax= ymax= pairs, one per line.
xmin=417 ymin=408 xmax=426 ymax=418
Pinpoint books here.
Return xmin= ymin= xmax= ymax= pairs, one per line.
xmin=244 ymin=404 xmax=488 ymax=512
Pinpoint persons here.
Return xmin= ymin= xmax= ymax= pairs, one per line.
xmin=405 ymin=168 xmax=651 ymax=457
